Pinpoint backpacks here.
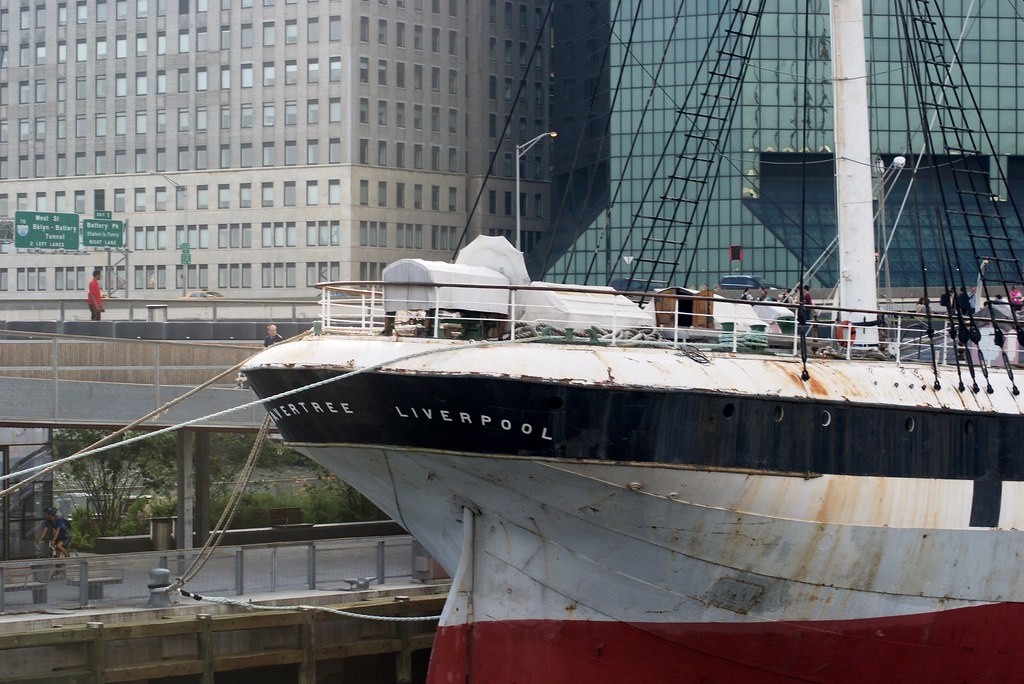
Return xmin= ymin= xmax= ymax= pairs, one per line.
xmin=741 ymin=294 xmax=748 ymax=300
xmin=940 ymin=293 xmax=951 ymax=306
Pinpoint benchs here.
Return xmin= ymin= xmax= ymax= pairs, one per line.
xmin=66 ymin=575 xmax=123 ymax=600
xmin=268 ymin=507 xmax=314 ymax=529
xmin=3 ymin=580 xmax=48 ymax=604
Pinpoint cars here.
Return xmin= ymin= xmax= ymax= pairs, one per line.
xmin=180 ymin=290 xmax=222 ymax=300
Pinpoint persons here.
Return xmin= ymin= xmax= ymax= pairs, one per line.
xmin=34 ymin=506 xmax=73 ymax=558
xmin=916 ymin=284 xmax=1022 ymax=314
xmin=263 ymin=324 xmax=283 ymax=347
xmin=740 ymin=284 xmax=812 ymax=320
xmin=87 ymin=270 xmax=105 ymax=321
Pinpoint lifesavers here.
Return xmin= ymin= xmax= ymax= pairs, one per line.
xmin=836 ymin=319 xmax=857 ymax=349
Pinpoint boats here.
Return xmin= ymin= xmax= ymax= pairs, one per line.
xmin=234 ymin=0 xmax=1024 ymax=682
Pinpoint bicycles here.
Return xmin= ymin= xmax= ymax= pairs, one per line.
xmin=32 ymin=542 xmax=80 ymax=583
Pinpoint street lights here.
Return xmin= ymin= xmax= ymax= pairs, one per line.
xmin=515 ymin=133 xmax=558 ymax=251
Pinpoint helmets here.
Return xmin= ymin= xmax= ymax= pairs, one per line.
xmin=44 ymin=507 xmax=57 ymax=516
